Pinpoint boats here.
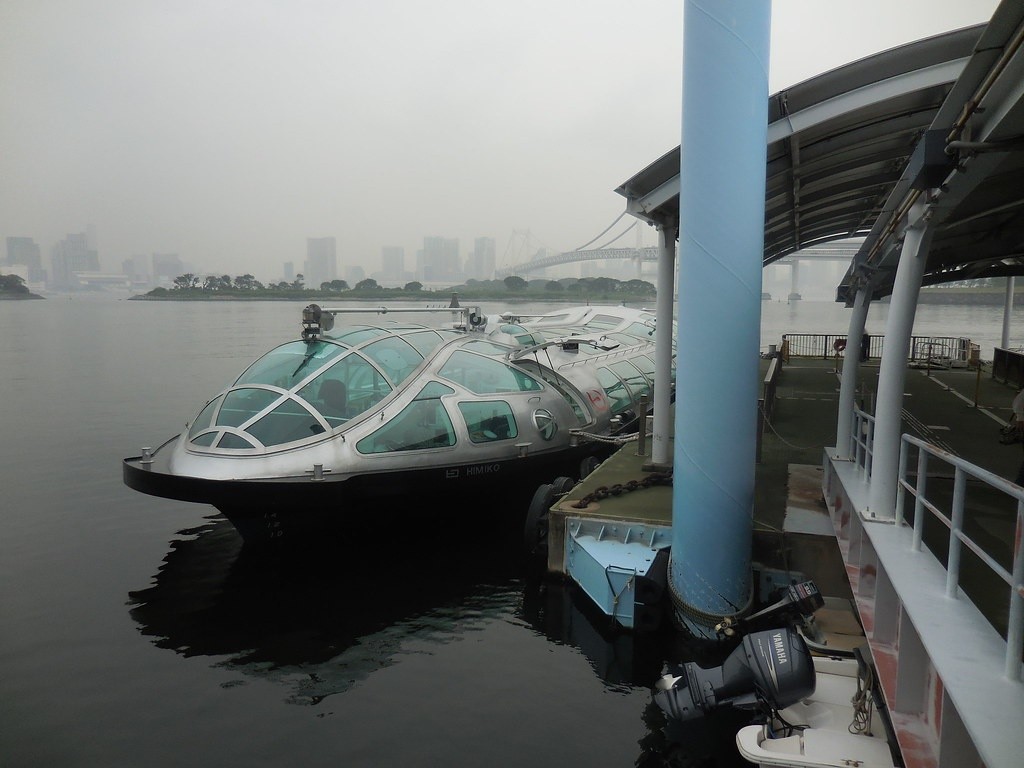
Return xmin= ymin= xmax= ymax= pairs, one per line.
xmin=122 ymin=298 xmax=679 ymax=549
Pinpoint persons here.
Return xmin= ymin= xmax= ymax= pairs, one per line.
xmin=859 ymin=328 xmax=870 ymax=361
